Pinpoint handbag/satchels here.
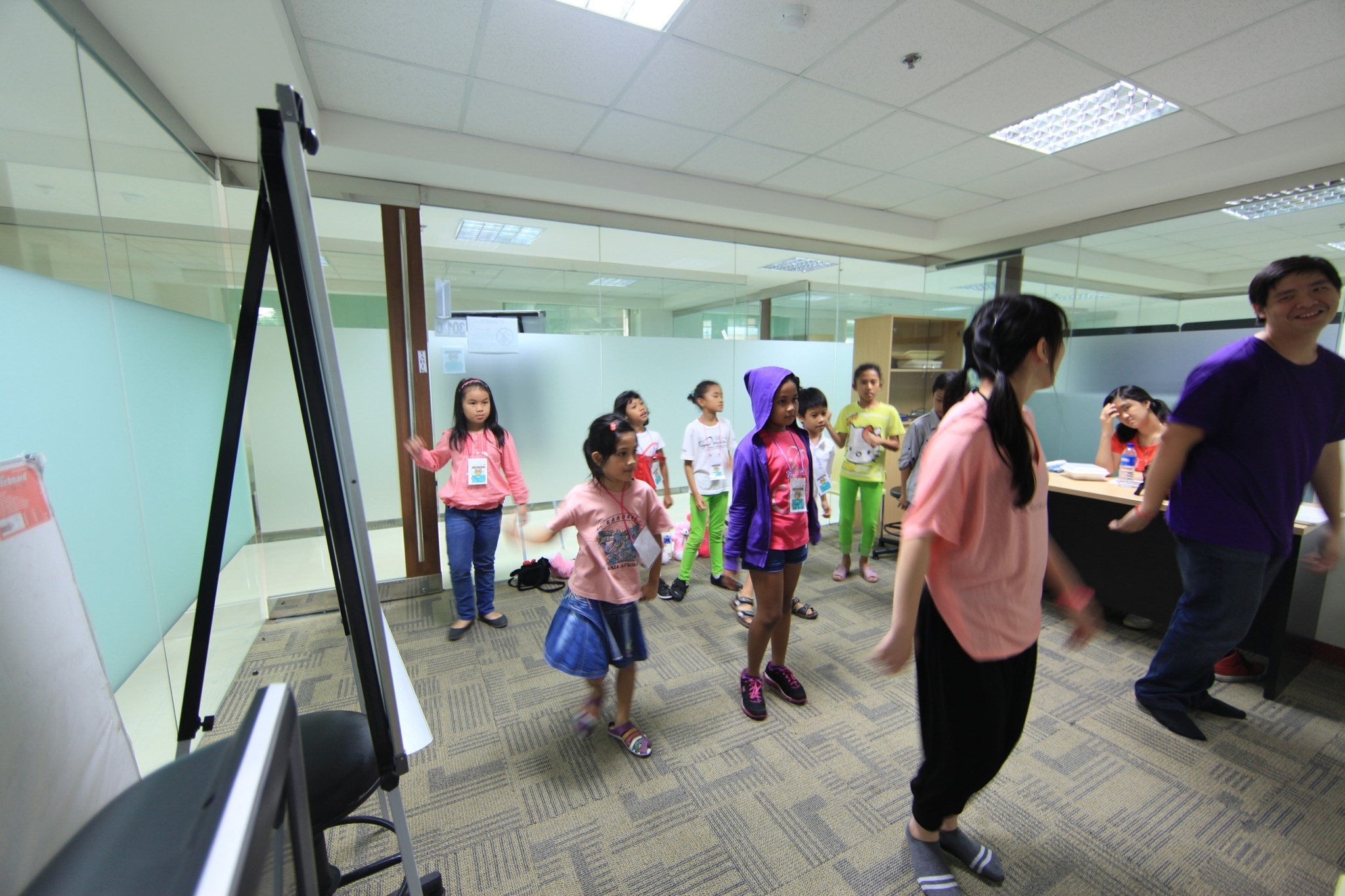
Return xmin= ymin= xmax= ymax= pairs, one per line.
xmin=508 ymin=559 xmax=565 ymax=593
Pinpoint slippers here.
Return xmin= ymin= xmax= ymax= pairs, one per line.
xmin=607 ymin=720 xmax=652 ymax=757
xmin=833 ymin=568 xmax=850 ymax=581
xmin=573 ymin=699 xmax=602 ymax=739
xmin=860 ymin=566 xmax=878 ymax=582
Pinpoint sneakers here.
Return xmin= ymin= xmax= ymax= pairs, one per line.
xmin=669 ymin=577 xmax=689 ymax=602
xmin=1211 ymin=648 xmax=1266 ymax=682
xmin=711 ymin=574 xmax=743 ymax=591
xmin=656 ymin=578 xmax=672 ymax=598
xmin=740 ymin=668 xmax=767 ymax=719
xmin=763 ymin=661 xmax=806 ymax=704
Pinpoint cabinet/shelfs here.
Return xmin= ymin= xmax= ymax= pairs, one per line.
xmin=851 ymin=313 xmax=967 ymax=535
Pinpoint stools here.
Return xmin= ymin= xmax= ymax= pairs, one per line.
xmin=871 ymin=486 xmax=901 ymax=560
xmin=293 ymin=707 xmax=446 ymax=896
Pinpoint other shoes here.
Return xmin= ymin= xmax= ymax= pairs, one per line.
xmin=448 ymin=620 xmax=473 ymax=639
xmin=480 ymin=612 xmax=508 ymax=627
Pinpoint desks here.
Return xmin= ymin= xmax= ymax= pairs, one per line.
xmin=1039 ymin=467 xmax=1333 ymax=699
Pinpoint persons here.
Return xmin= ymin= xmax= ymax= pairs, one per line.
xmin=728 ymin=389 xmax=831 ymax=631
xmin=615 ymin=391 xmax=678 ymax=600
xmin=825 ymin=363 xmax=907 ymax=580
xmin=1109 ymin=255 xmax=1345 ymax=741
xmin=668 ymin=380 xmax=747 ymax=602
xmin=1094 ymin=384 xmax=1187 ymax=629
xmin=897 ymin=371 xmax=970 ymax=512
xmin=499 ymin=413 xmax=674 ymax=755
xmin=721 ymin=366 xmax=822 ymax=721
xmin=403 ymin=378 xmax=529 ymax=641
xmin=868 ymin=292 xmax=1105 ymax=895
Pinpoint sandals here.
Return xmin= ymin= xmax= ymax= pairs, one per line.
xmin=791 ymin=597 xmax=818 ymax=619
xmin=730 ymin=594 xmax=755 ymax=628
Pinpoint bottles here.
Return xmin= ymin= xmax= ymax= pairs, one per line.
xmin=1118 ymin=442 xmax=1138 ymax=491
